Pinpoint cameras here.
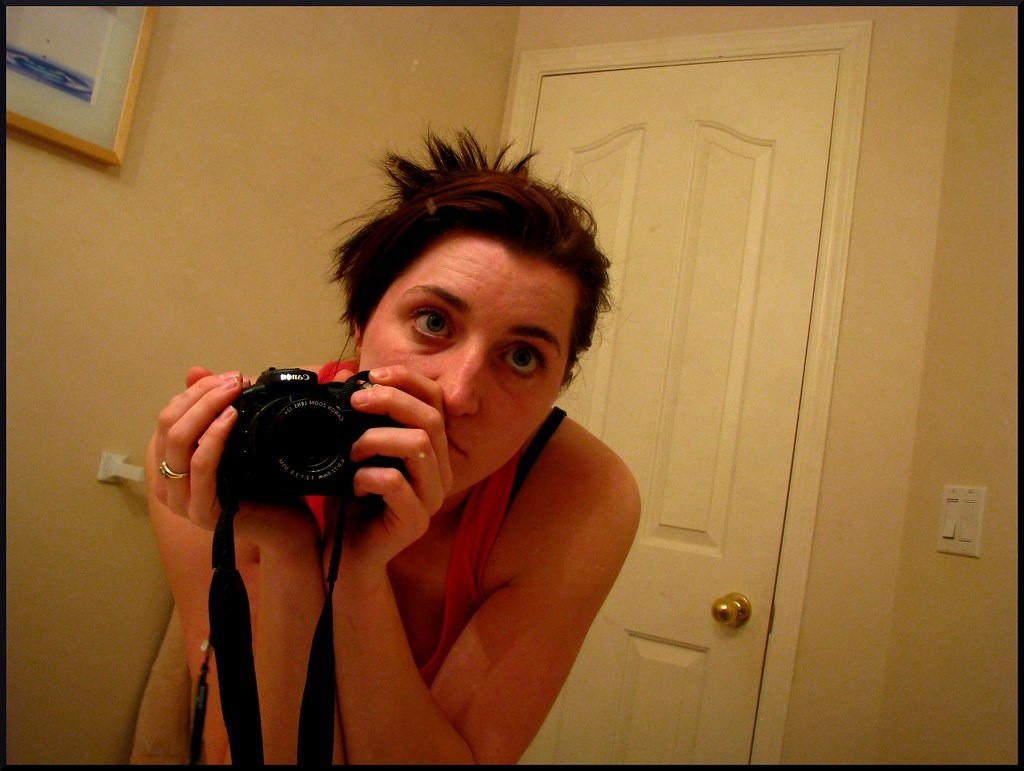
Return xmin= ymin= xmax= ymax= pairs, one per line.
xmin=212 ymin=366 xmax=403 ymax=500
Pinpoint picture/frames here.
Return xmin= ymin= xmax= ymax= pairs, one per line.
xmin=5 ymin=7 xmax=154 ymax=166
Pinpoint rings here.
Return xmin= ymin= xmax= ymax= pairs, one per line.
xmin=159 ymin=461 xmax=189 ymax=480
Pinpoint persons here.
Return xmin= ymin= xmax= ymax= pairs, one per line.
xmin=148 ymin=127 xmax=639 ymax=765
